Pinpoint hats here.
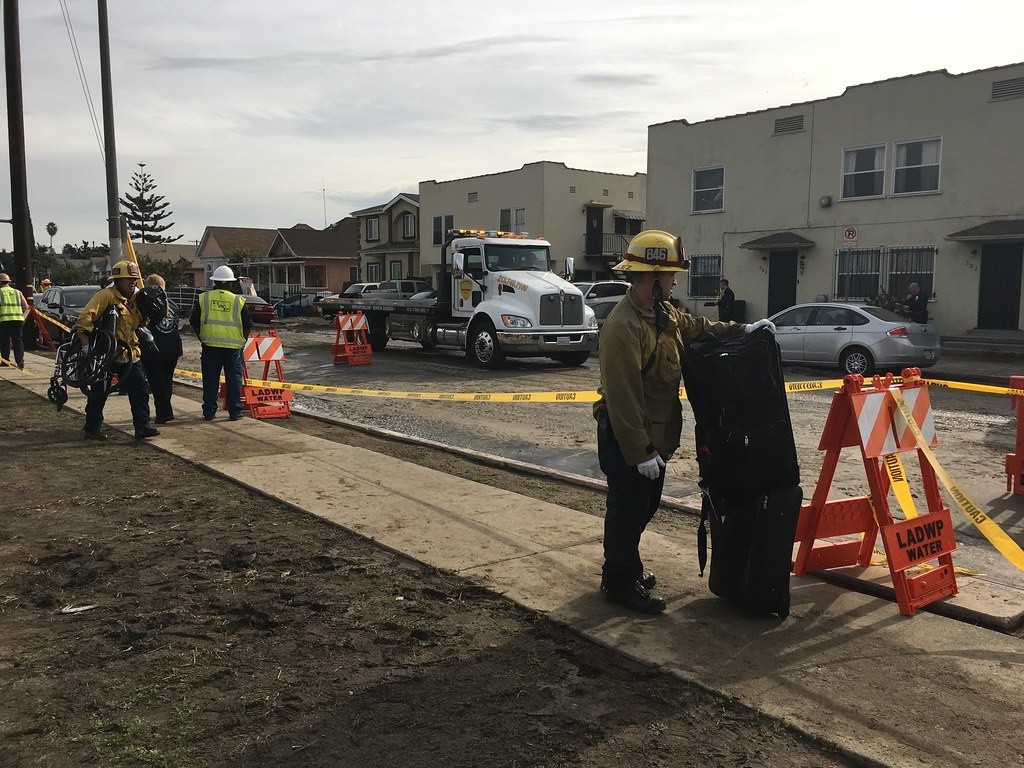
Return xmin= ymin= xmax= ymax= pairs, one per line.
xmin=146 ymin=273 xmax=165 ymax=290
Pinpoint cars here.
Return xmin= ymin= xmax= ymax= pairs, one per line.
xmin=766 ymin=302 xmax=942 ymax=377
xmin=236 ymin=295 xmax=276 ymax=324
xmin=318 ymin=283 xmax=380 ymax=318
xmin=32 ymin=285 xmax=107 ymax=349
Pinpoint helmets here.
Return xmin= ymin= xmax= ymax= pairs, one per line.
xmin=209 ymin=265 xmax=237 ymax=281
xmin=0 ymin=273 xmax=11 ymax=281
xmin=611 ymin=230 xmax=690 ymax=272
xmin=42 ymin=279 xmax=52 ymax=284
xmin=107 ymin=261 xmax=141 ymax=281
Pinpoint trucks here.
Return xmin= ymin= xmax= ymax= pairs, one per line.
xmin=313 ymin=227 xmax=601 ymax=370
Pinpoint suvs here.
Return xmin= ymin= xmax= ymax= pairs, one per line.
xmin=567 ymin=278 xmax=632 ymax=332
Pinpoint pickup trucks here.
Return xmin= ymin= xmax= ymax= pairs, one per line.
xmin=358 ymin=278 xmax=437 ymax=301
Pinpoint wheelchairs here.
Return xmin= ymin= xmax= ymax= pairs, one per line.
xmin=46 ymin=304 xmax=134 ymax=412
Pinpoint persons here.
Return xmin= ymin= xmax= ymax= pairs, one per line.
xmin=903 ymin=283 xmax=928 ymax=323
xmin=38 ymin=279 xmax=52 ymax=292
xmin=144 ymin=275 xmax=183 ymax=423
xmin=0 ymin=273 xmax=29 ymax=369
xmin=189 ymin=266 xmax=254 ymax=421
xmin=595 ymin=231 xmax=775 ymax=610
xmin=100 ymin=276 xmax=112 ymax=289
xmin=714 ymin=279 xmax=734 ymax=322
xmin=76 ymin=261 xmax=160 ymax=441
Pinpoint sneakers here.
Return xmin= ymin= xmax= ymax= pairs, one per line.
xmin=604 ymin=580 xmax=665 ymax=614
xmin=600 ymin=570 xmax=655 ymax=590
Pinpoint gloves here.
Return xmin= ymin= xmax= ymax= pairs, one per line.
xmin=637 ymin=454 xmax=665 ymax=480
xmin=745 ymin=318 xmax=777 ymax=335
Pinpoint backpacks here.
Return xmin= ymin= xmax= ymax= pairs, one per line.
xmin=697 ymin=485 xmax=802 ymax=618
xmin=681 ymin=333 xmax=799 ymax=491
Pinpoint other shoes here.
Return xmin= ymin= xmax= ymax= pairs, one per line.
xmin=85 ymin=431 xmax=107 ymax=441
xmin=135 ymin=427 xmax=160 ymax=439
xmin=18 ymin=362 xmax=24 ymax=368
xmin=230 ymin=412 xmax=243 ymax=421
xmin=155 ymin=414 xmax=174 ymax=423
xmin=0 ymin=363 xmax=9 ymax=366
xmin=205 ymin=412 xmax=215 ymax=421
